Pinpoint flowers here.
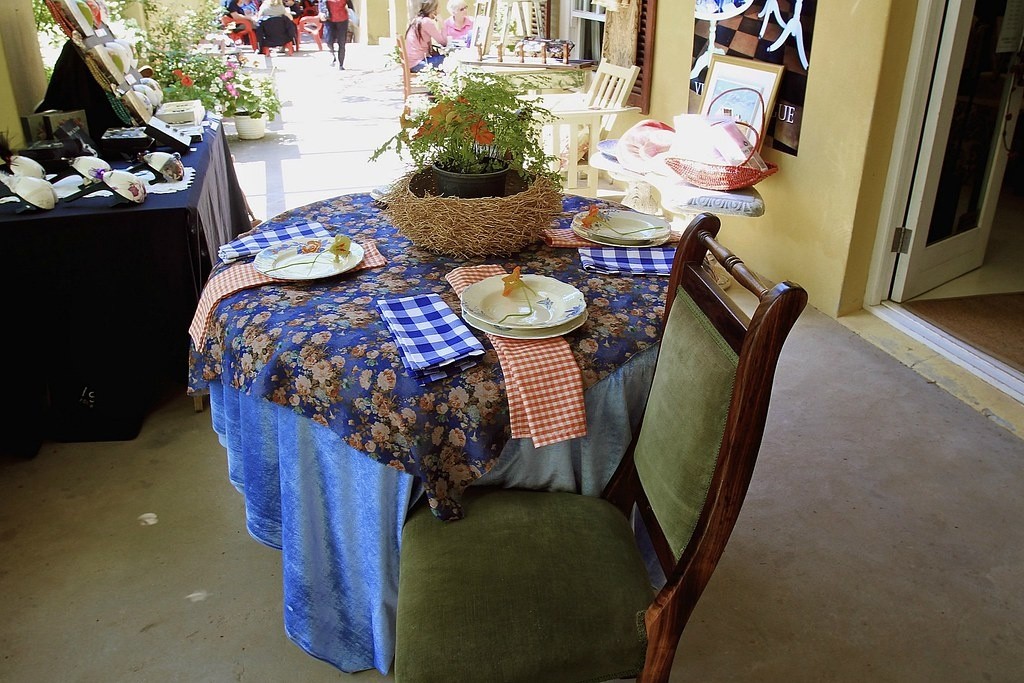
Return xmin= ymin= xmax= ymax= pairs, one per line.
xmin=108 ymin=0 xmax=280 ymax=122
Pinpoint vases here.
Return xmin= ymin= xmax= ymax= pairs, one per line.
xmin=235 ymin=111 xmax=266 ymax=140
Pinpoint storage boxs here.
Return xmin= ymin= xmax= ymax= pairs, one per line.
xmin=13 ymin=100 xmax=204 ymax=170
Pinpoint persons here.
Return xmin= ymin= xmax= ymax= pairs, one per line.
xmin=445 ymin=0 xmax=474 ymax=40
xmin=223 ymin=0 xmax=354 ymax=69
xmin=405 ymin=0 xmax=447 ymax=74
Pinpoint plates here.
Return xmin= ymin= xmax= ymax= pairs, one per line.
xmin=461 ymin=274 xmax=587 ymax=329
xmin=370 ymin=184 xmax=392 ymax=203
xmin=571 ymin=209 xmax=671 ymax=248
xmin=252 ymin=237 xmax=364 ymax=280
xmin=461 ymin=309 xmax=589 ymax=339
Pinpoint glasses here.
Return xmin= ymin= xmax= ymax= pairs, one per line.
xmin=456 ymin=5 xmax=468 ymax=11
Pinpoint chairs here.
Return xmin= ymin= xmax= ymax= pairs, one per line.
xmin=396 ymin=35 xmax=430 ymax=101
xmin=221 ymin=0 xmax=326 ymax=56
xmin=393 ymin=213 xmax=806 ymax=683
xmin=534 ymin=56 xmax=641 ymax=205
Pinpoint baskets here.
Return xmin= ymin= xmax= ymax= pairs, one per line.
xmin=664 ymin=87 xmax=778 ymax=191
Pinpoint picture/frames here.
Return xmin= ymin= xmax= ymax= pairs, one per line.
xmin=696 ymin=54 xmax=785 ymax=156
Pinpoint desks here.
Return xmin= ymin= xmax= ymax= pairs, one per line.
xmin=0 ymin=119 xmax=258 ymax=461
xmin=186 ymin=194 xmax=685 ymax=674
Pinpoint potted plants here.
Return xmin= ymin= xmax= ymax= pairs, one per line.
xmin=372 ymin=65 xmax=585 ymax=200
